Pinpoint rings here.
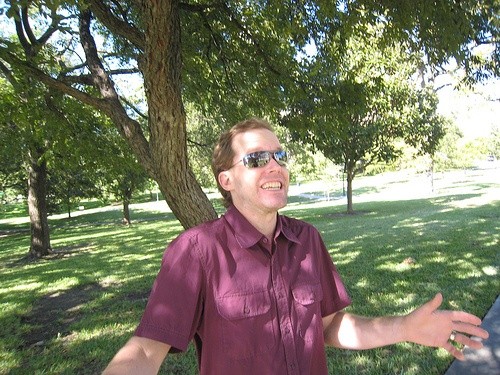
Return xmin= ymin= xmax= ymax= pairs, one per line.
xmin=449 ymin=331 xmax=456 ymax=341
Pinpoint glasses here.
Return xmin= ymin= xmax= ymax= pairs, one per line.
xmin=227 ymin=150 xmax=289 ymax=169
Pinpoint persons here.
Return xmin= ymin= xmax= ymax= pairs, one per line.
xmin=101 ymin=116 xmax=489 ymax=375
